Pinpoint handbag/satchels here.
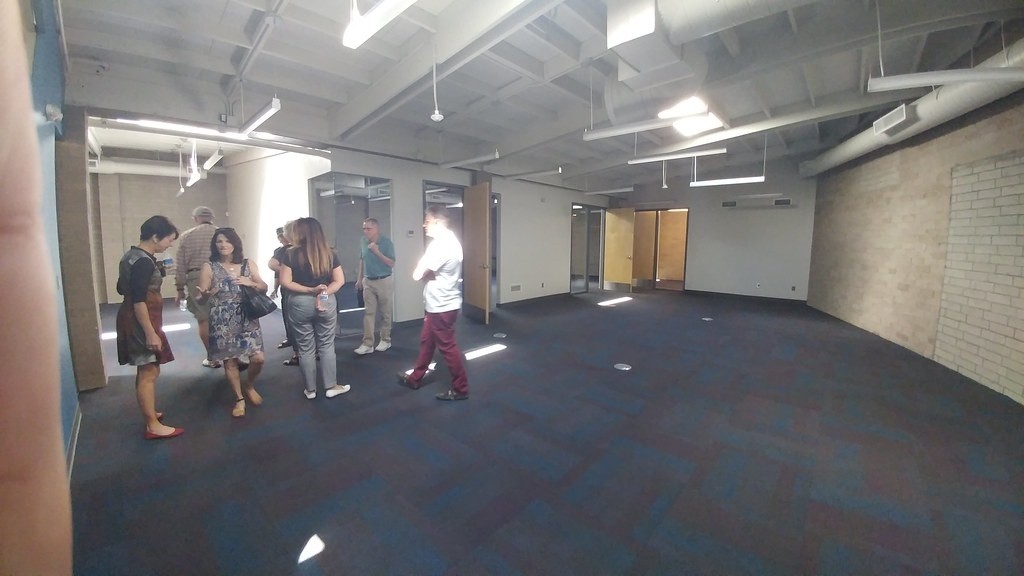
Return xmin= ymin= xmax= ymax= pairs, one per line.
xmin=357 ymin=288 xmax=365 ymax=307
xmin=239 ymin=258 xmax=277 ymax=321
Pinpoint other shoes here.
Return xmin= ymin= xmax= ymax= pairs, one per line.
xmin=157 ymin=412 xmax=164 ymax=421
xmin=232 ymin=397 xmax=246 ymax=418
xmin=435 ymin=389 xmax=470 ymax=401
xmin=144 ymin=426 xmax=186 ymax=440
xmin=202 ymin=358 xmax=221 ymax=368
xmin=244 ymin=386 xmax=263 ymax=405
xmin=303 ymin=388 xmax=317 ymax=399
xmin=278 ymin=338 xmax=291 ymax=349
xmin=397 ymin=370 xmax=420 ymax=390
xmin=282 ymin=355 xmax=299 ymax=366
xmin=326 ymin=384 xmax=350 ymax=398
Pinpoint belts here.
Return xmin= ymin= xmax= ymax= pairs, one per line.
xmin=368 ymin=274 xmax=391 ymax=280
xmin=186 ymin=267 xmax=202 ymax=272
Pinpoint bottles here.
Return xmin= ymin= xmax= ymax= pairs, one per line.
xmin=163 ymin=250 xmax=175 ymax=275
xmin=320 ymin=284 xmax=327 ymax=310
xmin=229 ymin=268 xmax=241 ymax=293
xmin=180 ymin=297 xmax=187 ymax=311
xmin=356 ymin=289 xmax=365 ymax=307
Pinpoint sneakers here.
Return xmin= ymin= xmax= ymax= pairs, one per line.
xmin=375 ymin=340 xmax=392 ymax=351
xmin=353 ymin=344 xmax=374 ymax=354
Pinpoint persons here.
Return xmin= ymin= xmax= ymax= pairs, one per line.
xmin=174 ymin=206 xmax=221 ymax=368
xmin=116 ymin=216 xmax=185 ymax=440
xmin=268 ymin=217 xmax=351 ymax=399
xmin=195 ymin=227 xmax=268 ymax=417
xmin=397 ymin=205 xmax=470 ymax=401
xmin=354 ymin=217 xmax=396 ymax=354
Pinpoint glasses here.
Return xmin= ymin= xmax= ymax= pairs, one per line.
xmin=424 ymin=219 xmax=437 ymax=225
xmin=361 ymin=227 xmax=376 ymax=232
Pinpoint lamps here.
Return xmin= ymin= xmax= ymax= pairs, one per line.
xmin=45 ymin=104 xmax=63 ymax=122
xmin=583 ymin=59 xmax=767 ymax=196
xmin=343 ymin=0 xmax=420 ymax=49
xmin=240 ymin=79 xmax=281 ymax=137
xmin=867 ymin=0 xmax=1024 ymax=93
xmin=439 ymin=131 xmax=500 ymax=170
xmin=431 ymin=39 xmax=444 ymax=122
xmin=174 ymin=138 xmax=225 ymax=197
xmin=503 ymin=166 xmax=561 ymax=182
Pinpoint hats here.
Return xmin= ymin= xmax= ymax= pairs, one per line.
xmin=277 ymin=227 xmax=283 ymax=238
xmin=192 ymin=206 xmax=215 ymax=217
